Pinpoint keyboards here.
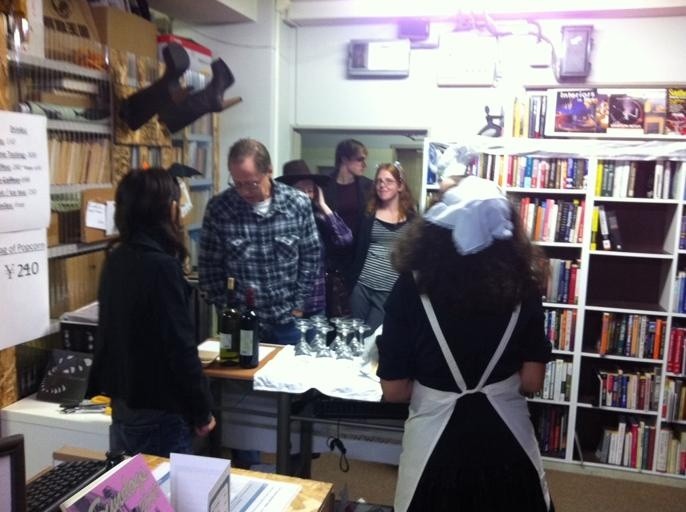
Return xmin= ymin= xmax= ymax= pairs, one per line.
xmin=26 ymin=460 xmax=113 ymax=512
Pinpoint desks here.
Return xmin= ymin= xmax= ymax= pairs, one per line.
xmin=29 ymin=449 xmax=331 ymax=512
xmin=195 ymin=333 xmax=405 ymax=475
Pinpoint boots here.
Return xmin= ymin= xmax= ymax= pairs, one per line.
xmin=162 ymin=56 xmax=243 ymax=135
xmin=119 ymin=41 xmax=196 ymax=132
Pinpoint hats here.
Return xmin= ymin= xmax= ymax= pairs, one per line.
xmin=273 ymin=159 xmax=332 ymax=187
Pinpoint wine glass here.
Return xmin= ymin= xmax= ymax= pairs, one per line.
xmin=294 ymin=314 xmax=371 ymax=361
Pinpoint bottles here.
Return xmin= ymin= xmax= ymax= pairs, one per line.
xmin=240 ymin=288 xmax=258 ymax=368
xmin=219 ymin=277 xmax=240 ymax=360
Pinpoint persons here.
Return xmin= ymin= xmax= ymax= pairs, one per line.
xmin=321 ymin=138 xmax=375 ymax=347
xmin=375 ymin=176 xmax=555 ymax=512
xmin=275 ymin=159 xmax=353 ymax=320
xmin=478 ymin=106 xmax=503 ymax=137
xmin=84 ymin=166 xmax=217 ymax=457
xmin=197 ymin=137 xmax=323 ymax=466
xmin=344 ymin=163 xmax=421 ymax=338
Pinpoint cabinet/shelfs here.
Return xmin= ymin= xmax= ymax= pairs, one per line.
xmin=422 ymin=136 xmax=686 ymax=488
xmin=1 ymin=25 xmax=218 ymax=405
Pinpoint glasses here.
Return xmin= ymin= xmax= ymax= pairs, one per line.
xmin=227 ymin=174 xmax=266 ymax=190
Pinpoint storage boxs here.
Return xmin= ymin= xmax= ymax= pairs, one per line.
xmin=92 ymin=6 xmax=159 ymax=61
xmin=156 ymin=33 xmax=212 ymax=75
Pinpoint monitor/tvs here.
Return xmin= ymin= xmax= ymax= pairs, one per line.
xmin=0 ymin=435 xmax=26 ymax=512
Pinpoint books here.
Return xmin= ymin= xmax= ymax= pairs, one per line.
xmin=16 ymin=70 xmax=217 ymax=400
xmin=520 ymin=197 xmax=586 ymax=243
xmin=597 ymin=313 xmax=667 ymax=360
xmin=537 ymin=407 xmax=569 ymax=459
xmin=590 ymin=206 xmax=623 ymax=251
xmin=426 ymin=192 xmax=440 ymax=210
xmin=595 ymin=160 xmax=682 ymax=200
xmin=656 ymin=428 xmax=686 ymax=476
xmin=662 ymin=378 xmax=685 ymax=423
xmin=541 ymin=258 xmax=582 ymax=305
xmin=528 ymin=359 xmax=573 ymax=402
xmin=594 ymin=416 xmax=656 ymax=470
xmin=496 ymin=87 xmax=685 ymax=139
xmin=595 ymin=365 xmax=662 ymax=412
xmin=59 ymin=452 xmax=175 ymax=512
xmin=544 ymin=309 xmax=578 ymax=352
xmin=465 ymin=153 xmax=588 ymax=189
xmin=673 ymin=216 xmax=685 ymax=313
xmin=666 ymin=328 xmax=685 ymax=374
